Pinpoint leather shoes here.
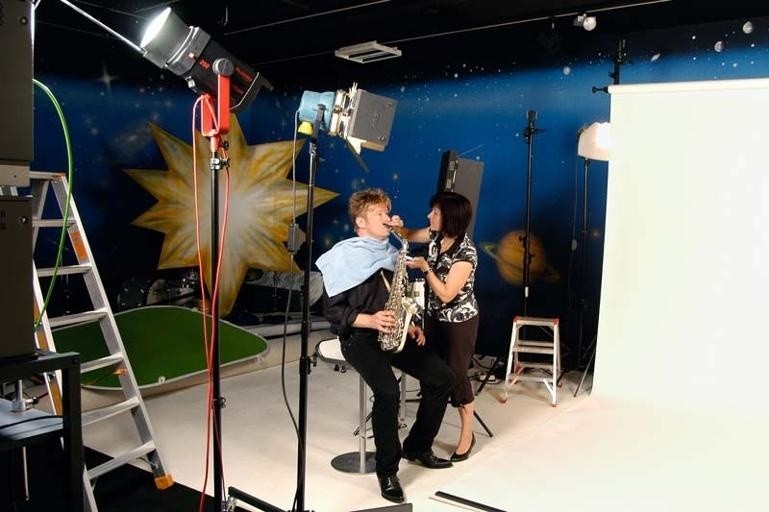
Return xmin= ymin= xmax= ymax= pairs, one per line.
xmin=401 ymin=436 xmax=453 ymax=468
xmin=450 ymin=429 xmax=478 ymax=463
xmin=376 ymin=467 xmax=407 ymax=503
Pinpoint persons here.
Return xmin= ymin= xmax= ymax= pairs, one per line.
xmin=324 ymin=188 xmax=453 ymax=503
xmin=388 ymin=192 xmax=480 ymax=463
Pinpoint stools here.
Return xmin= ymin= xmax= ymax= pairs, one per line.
xmin=316 ymin=336 xmax=396 ymax=474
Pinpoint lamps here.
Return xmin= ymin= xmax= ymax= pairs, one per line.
xmin=137 ymin=7 xmax=261 ymax=508
xmin=290 ymin=84 xmax=400 ymax=512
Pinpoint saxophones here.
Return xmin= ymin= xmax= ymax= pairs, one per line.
xmin=378 ymin=223 xmax=423 ymax=352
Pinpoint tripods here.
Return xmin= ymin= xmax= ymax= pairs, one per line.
xmin=229 ymin=142 xmax=413 ymax=512
xmin=354 ymin=273 xmax=494 ymax=440
xmin=565 ymin=160 xmax=594 ymax=375
xmin=474 ymin=122 xmax=575 ymax=397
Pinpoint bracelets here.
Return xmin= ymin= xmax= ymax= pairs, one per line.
xmin=421 ymin=267 xmax=434 ymax=276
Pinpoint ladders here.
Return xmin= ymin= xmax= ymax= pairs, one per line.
xmin=1 ymin=171 xmax=174 ymax=512
